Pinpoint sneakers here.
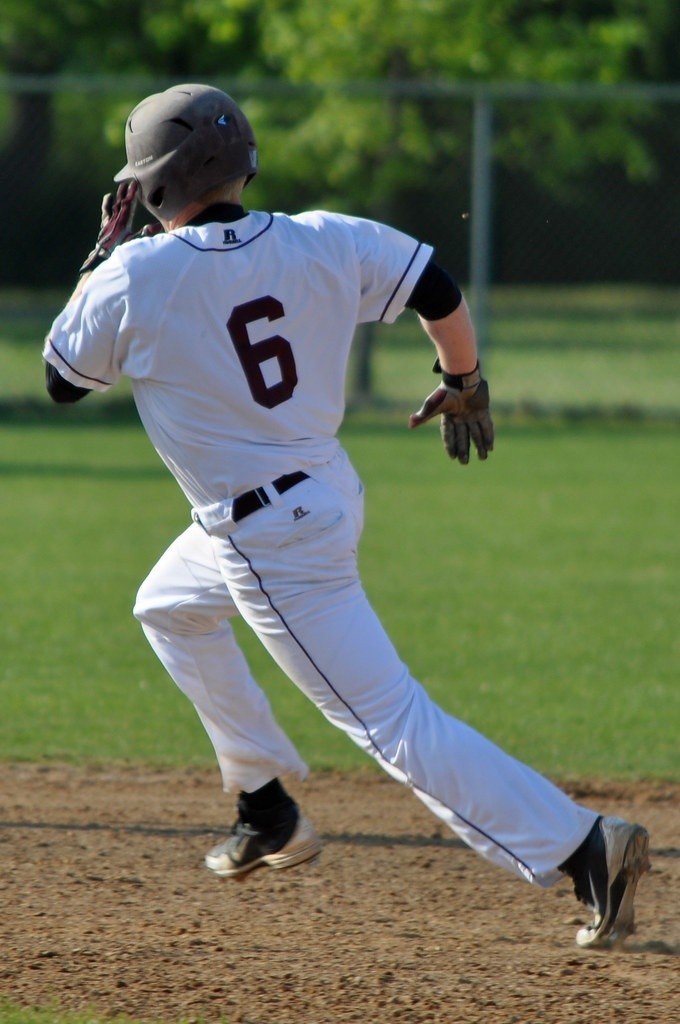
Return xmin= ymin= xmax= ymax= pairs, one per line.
xmin=205 ymin=792 xmax=323 ymax=880
xmin=567 ymin=813 xmax=651 ymax=950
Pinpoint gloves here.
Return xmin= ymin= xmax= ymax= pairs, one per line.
xmin=81 ymin=179 xmax=155 ymax=270
xmin=407 ymin=362 xmax=496 ymax=466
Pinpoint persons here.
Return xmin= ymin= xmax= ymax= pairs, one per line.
xmin=44 ymin=84 xmax=650 ymax=949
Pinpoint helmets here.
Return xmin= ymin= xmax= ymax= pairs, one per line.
xmin=114 ymin=83 xmax=256 ymax=222
